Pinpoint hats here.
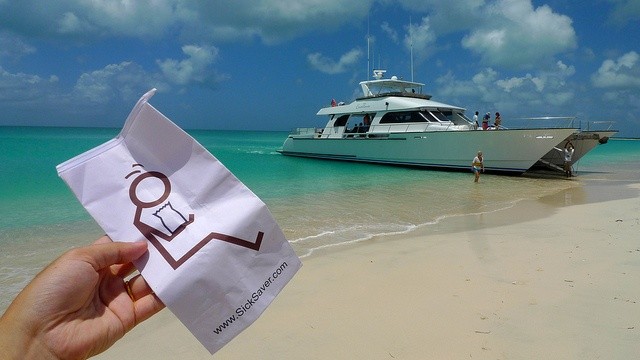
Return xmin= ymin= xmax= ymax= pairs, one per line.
xmin=495 ymin=112 xmax=500 ymax=115
xmin=486 ymin=112 xmax=490 ymax=115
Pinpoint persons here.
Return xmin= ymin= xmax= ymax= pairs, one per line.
xmin=0 ymin=233 xmax=168 ymax=360
xmin=348 ymin=114 xmax=370 ymax=136
xmin=482 ymin=112 xmax=491 ymax=130
xmin=494 ymin=112 xmax=501 ymax=130
xmin=563 ymin=141 xmax=575 ymax=178
xmin=472 ymin=149 xmax=485 ymax=183
xmin=472 ymin=111 xmax=479 ymax=130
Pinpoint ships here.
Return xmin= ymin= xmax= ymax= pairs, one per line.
xmin=277 ymin=15 xmax=620 ymax=174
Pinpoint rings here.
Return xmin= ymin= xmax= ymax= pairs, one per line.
xmin=124 ymin=280 xmax=135 ymax=302
